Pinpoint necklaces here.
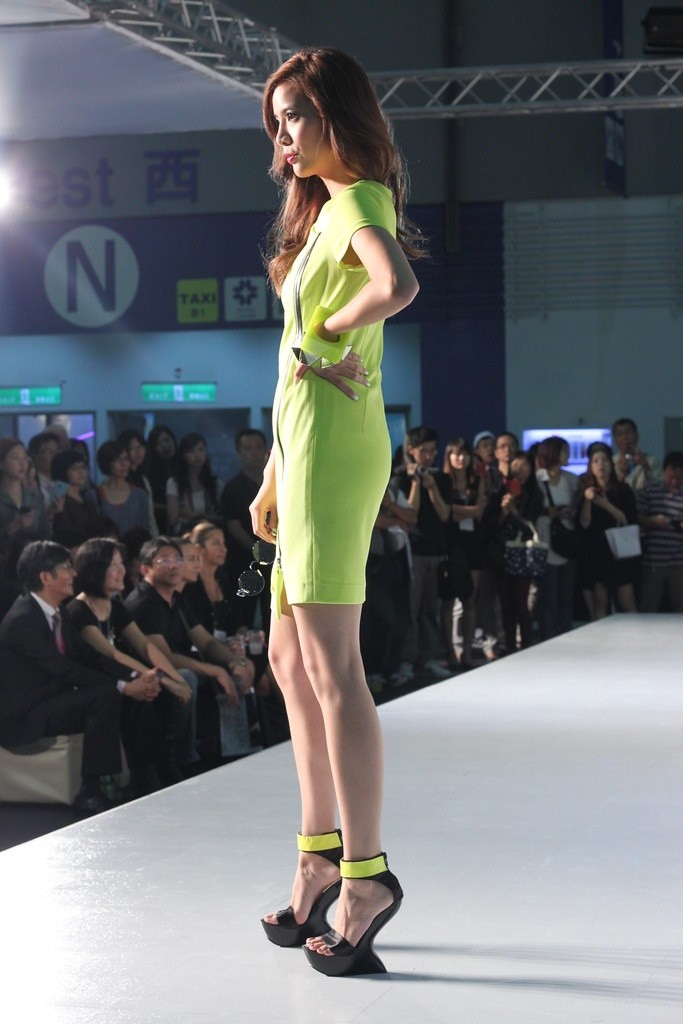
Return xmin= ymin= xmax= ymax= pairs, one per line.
xmin=92 ymin=602 xmax=110 ymax=637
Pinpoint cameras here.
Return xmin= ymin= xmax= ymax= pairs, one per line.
xmin=415 ymin=466 xmax=440 ymax=477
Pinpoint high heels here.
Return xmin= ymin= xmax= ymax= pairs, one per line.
xmin=301 ymin=850 xmax=404 ymax=976
xmin=260 ymin=828 xmax=345 ymax=948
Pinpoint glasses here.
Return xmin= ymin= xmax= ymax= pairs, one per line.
xmin=236 ymin=529 xmax=277 ymax=597
xmin=147 ymin=558 xmax=185 ymax=567
xmin=49 ymin=562 xmax=72 ymax=570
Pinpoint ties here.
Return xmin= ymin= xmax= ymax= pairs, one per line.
xmin=52 ymin=611 xmax=65 ymax=655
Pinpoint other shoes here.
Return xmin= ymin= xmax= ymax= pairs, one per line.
xmin=73 ymin=789 xmax=114 ymax=815
xmin=365 ymin=632 xmax=536 ymax=693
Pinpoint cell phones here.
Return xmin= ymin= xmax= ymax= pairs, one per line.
xmin=19 ymin=505 xmax=31 ymax=515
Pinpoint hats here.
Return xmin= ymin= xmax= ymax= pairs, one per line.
xmin=473 ymin=431 xmax=494 ymax=446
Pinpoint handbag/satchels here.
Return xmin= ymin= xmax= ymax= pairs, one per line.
xmin=505 ymin=520 xmax=548 ymax=577
xmin=549 ymin=517 xmax=576 ymax=558
xmin=605 ymin=515 xmax=641 ymax=558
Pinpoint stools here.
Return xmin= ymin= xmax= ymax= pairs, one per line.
xmin=0 ymin=732 xmax=86 ymax=806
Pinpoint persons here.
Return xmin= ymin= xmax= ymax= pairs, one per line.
xmin=247 ymin=48 xmax=418 ymax=976
xmin=360 ymin=417 xmax=683 ymax=694
xmin=0 ymin=425 xmax=290 ymax=801
